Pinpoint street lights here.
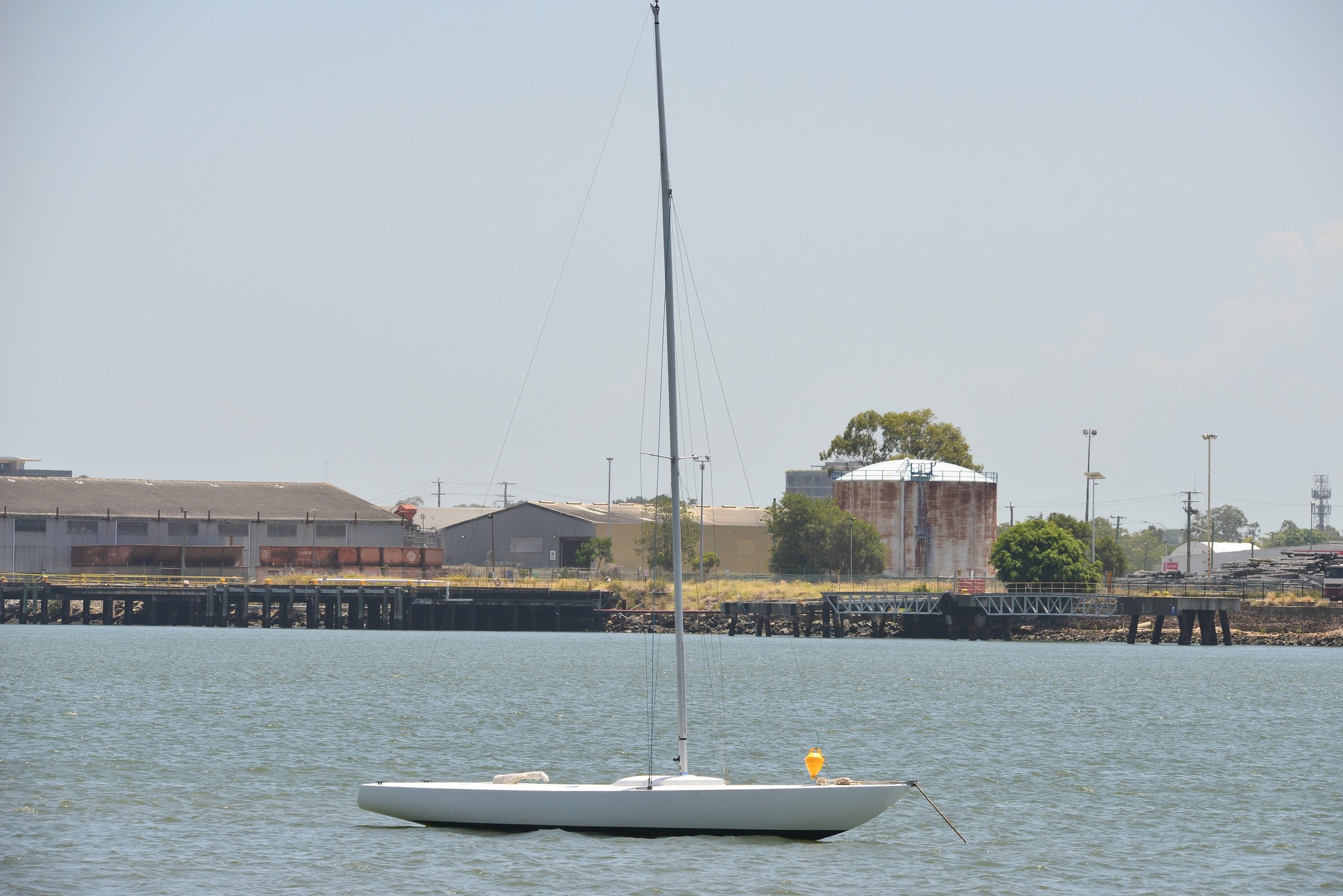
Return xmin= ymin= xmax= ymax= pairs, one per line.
xmin=1083 ymin=428 xmax=1097 ymax=525
xmin=1141 ymin=520 xmax=1168 ymax=573
xmin=606 ymin=457 xmax=613 ymax=564
xmin=419 ymin=513 xmax=427 ymax=549
xmin=310 ymin=509 xmax=318 ymax=574
xmin=487 ymin=513 xmax=496 ymax=581
xmin=1310 ymin=501 xmax=1317 ymax=553
xmin=1210 ymin=516 xmax=1217 ymax=571
xmin=179 ymin=507 xmax=187 ymax=587
xmin=1201 ymin=433 xmax=1218 ymax=580
xmin=691 ymin=453 xmax=712 ymax=583
xmin=846 ymin=516 xmax=856 ymax=585
xmin=1084 ymin=471 xmax=1106 ymax=567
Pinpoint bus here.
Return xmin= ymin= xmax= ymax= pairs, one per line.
xmin=1323 ymin=563 xmax=1343 ymax=601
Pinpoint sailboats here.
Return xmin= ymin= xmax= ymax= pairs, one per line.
xmin=356 ymin=1 xmax=923 ymax=835
